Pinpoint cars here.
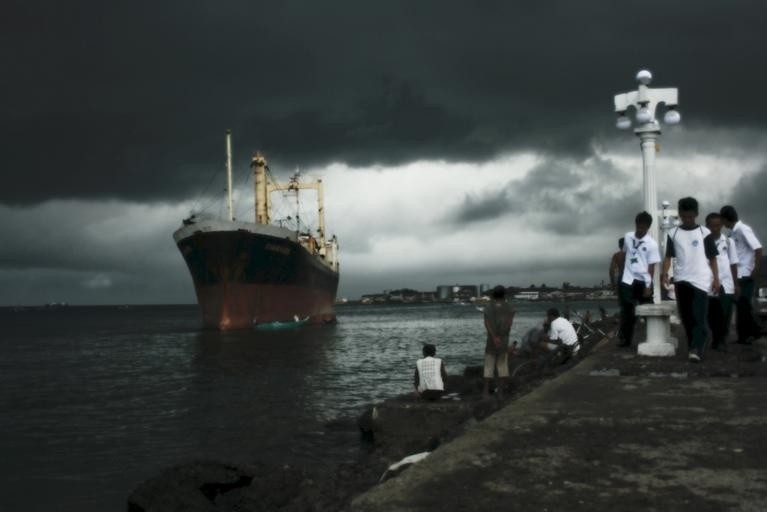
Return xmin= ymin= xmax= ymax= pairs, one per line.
xmin=752 ymin=286 xmax=767 ymax=321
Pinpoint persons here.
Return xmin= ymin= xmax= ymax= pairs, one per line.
xmin=608 ymin=196 xmax=763 ymax=362
xmin=482 ymin=286 xmax=515 ymax=400
xmin=414 ymin=344 xmax=447 ymax=401
xmin=539 ymin=308 xmax=580 ymax=360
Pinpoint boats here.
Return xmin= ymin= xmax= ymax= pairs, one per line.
xmin=173 ymin=127 xmax=340 ymax=330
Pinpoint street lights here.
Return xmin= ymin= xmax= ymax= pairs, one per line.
xmin=612 ymin=68 xmax=682 ymax=357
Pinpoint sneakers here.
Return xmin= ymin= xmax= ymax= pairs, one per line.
xmin=688 ymin=351 xmax=703 ymax=362
